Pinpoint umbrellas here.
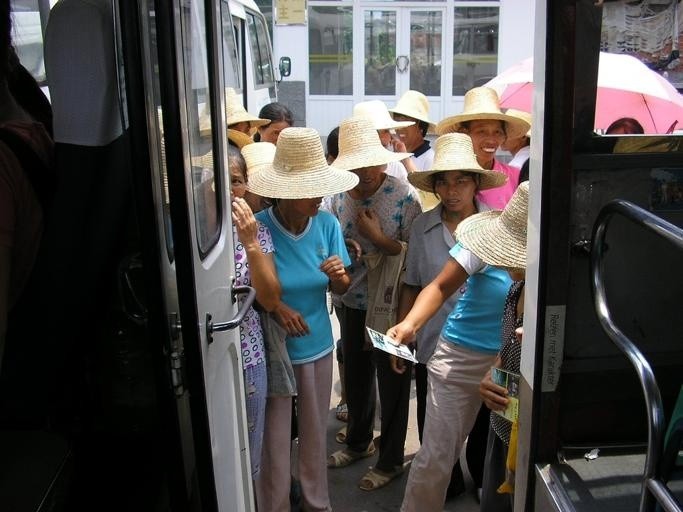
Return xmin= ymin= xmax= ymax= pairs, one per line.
xmin=479 ymin=49 xmax=683 ymax=136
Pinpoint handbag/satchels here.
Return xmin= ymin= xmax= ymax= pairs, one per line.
xmin=261 ymin=313 xmax=298 ymax=399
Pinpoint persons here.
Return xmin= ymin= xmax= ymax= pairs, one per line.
xmin=602 ymin=117 xmax=645 ymax=135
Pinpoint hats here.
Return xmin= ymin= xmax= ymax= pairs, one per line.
xmin=240 ymin=117 xmax=414 ymax=200
xmin=199 ymin=87 xmax=272 ymax=150
xmin=454 ymin=180 xmax=529 ymax=271
xmin=408 ymin=132 xmax=509 ymax=191
xmin=352 ymin=90 xmax=530 ymax=141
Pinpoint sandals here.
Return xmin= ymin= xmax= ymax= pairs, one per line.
xmin=325 ymin=439 xmax=376 ymax=468
xmin=360 ymin=471 xmax=391 ymax=491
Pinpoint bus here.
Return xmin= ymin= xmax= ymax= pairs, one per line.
xmin=307 ymin=12 xmax=353 ymax=97
xmin=379 ymin=14 xmax=498 ymax=91
xmin=9 ymin=0 xmax=290 ymax=133
